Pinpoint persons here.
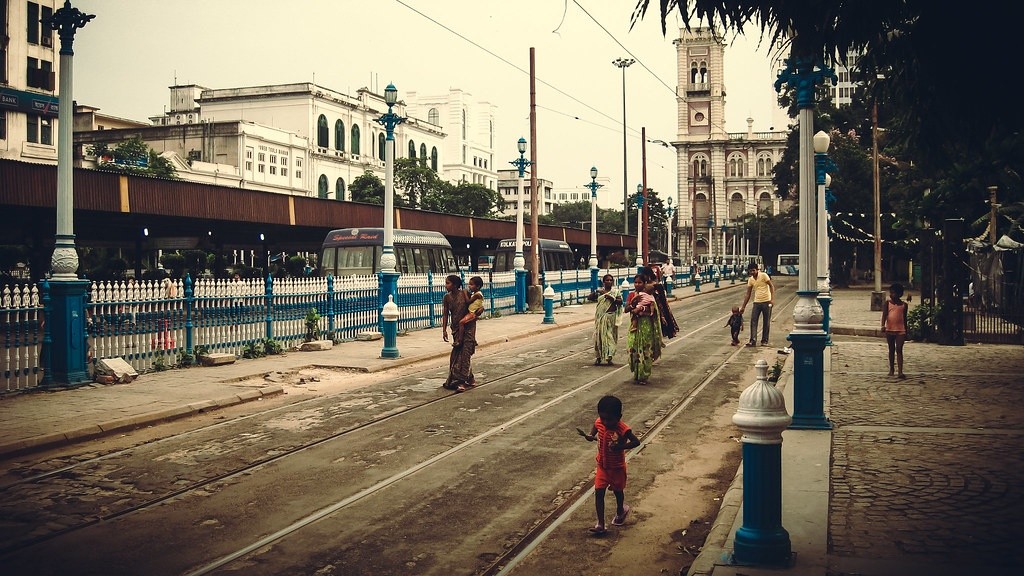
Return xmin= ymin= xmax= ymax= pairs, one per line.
xmin=231 ymin=274 xmax=241 ymax=283
xmin=739 ymin=264 xmax=775 ymax=346
xmin=624 ymin=268 xmax=674 ymax=384
xmin=442 ymin=275 xmax=484 ymax=391
xmin=576 ymin=395 xmax=641 ymax=534
xmin=724 ymin=306 xmax=744 ymax=345
xmin=881 ymin=283 xmax=909 ymax=379
xmin=650 ymin=258 xmax=675 ymax=291
xmin=766 ymin=263 xmax=772 ymax=280
xmin=587 ymin=274 xmax=623 ymax=366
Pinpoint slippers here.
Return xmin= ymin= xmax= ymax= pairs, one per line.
xmin=468 ymin=380 xmax=475 ymax=386
xmin=612 ymin=504 xmax=629 ymax=526
xmin=457 ymin=386 xmax=465 ymax=392
xmin=589 ymin=525 xmax=607 ymax=533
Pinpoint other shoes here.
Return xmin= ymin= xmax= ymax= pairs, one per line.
xmin=761 ymin=340 xmax=768 ymax=345
xmin=731 ymin=340 xmax=740 ymax=346
xmin=746 ymin=340 xmax=756 ymax=346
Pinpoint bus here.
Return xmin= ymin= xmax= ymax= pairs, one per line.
xmin=777 ymin=253 xmax=800 ymax=276
xmin=696 ymin=253 xmax=765 ymax=272
xmin=315 ymin=227 xmax=461 ymax=281
xmin=494 ymin=237 xmax=577 ymax=273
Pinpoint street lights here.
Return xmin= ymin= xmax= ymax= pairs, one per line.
xmin=627 ymin=183 xmax=650 ymax=266
xmin=507 ymin=135 xmax=536 ymax=269
xmin=663 ymin=196 xmax=677 ymax=264
xmin=611 ymin=57 xmax=637 ymax=264
xmin=370 ymin=80 xmax=409 ymax=272
xmin=706 ymin=212 xmax=714 ymax=265
xmin=720 ymin=215 xmax=730 ymax=265
xmin=820 ymin=171 xmax=834 ymax=297
xmin=581 ymin=165 xmax=605 ymax=268
xmin=814 ymin=129 xmax=834 ymax=349
